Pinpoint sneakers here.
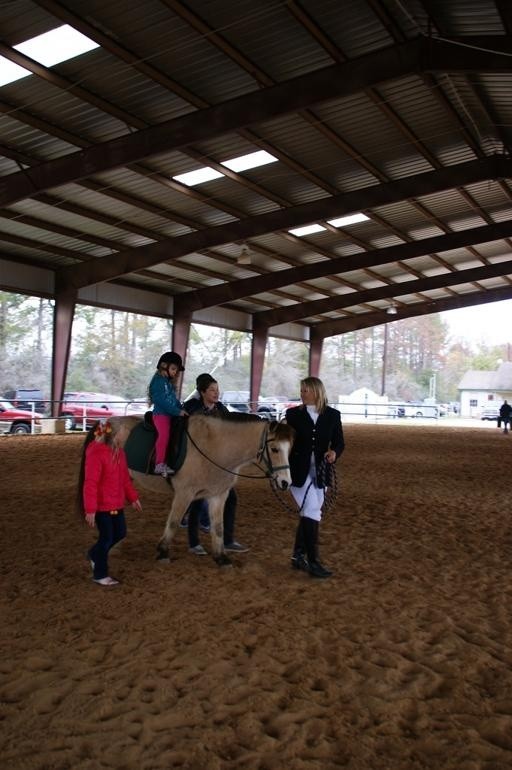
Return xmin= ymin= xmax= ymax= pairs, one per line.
xmin=93 ymin=575 xmax=119 ymax=586
xmin=180 ymin=512 xmax=188 ymax=526
xmin=189 ymin=543 xmax=207 ymax=555
xmin=89 ymin=549 xmax=96 ymax=570
xmin=223 ymin=540 xmax=250 ymax=553
xmin=154 ymin=463 xmax=175 ymax=473
xmin=198 ymin=519 xmax=210 ymax=532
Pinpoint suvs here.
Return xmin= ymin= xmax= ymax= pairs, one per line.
xmin=62 ymin=392 xmax=137 ymax=430
xmin=4 ymin=389 xmax=47 ymax=413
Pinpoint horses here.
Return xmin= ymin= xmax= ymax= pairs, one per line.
xmin=76 ymin=409 xmax=295 ymax=574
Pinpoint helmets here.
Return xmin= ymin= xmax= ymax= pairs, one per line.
xmin=157 ymin=352 xmax=185 ymax=371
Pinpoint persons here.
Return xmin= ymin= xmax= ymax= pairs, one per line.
xmin=281 ymin=377 xmax=344 ymax=578
xmin=180 ymin=373 xmax=250 ymax=556
xmin=500 ymin=400 xmax=512 ymax=433
xmin=178 ymin=498 xmax=210 ymax=533
xmin=147 ymin=351 xmax=189 ymax=475
xmin=82 ymin=420 xmax=142 ymax=586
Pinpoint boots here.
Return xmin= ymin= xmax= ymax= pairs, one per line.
xmin=304 ymin=516 xmax=332 ymax=579
xmin=290 ymin=516 xmax=310 ymax=572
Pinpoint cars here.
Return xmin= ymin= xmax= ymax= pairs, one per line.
xmin=220 ymin=391 xmax=276 ymax=416
xmin=482 ymin=405 xmax=500 ymax=420
xmin=0 ymin=397 xmax=42 ymax=434
xmin=399 ymin=401 xmax=445 ymax=416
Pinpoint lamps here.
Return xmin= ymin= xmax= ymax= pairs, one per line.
xmin=386 ymin=303 xmax=397 ymax=314
xmin=236 ymin=248 xmax=252 ymax=265
